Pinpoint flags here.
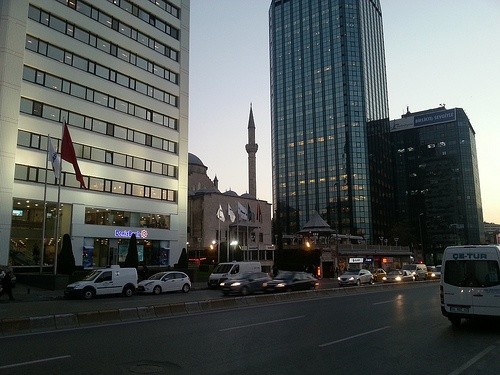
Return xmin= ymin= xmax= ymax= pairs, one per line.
xmin=238 ymin=202 xmax=248 ymax=220
xmin=216 ymin=204 xmax=226 ymax=222
xmin=248 ymin=203 xmax=255 ymax=221
xmin=60 ymin=119 xmax=86 ymax=190
xmin=228 ymin=203 xmax=236 ymax=222
xmin=255 ymin=203 xmax=263 ymax=223
xmin=47 ymin=138 xmax=60 ymax=179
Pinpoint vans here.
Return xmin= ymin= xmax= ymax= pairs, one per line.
xmin=209 ymin=261 xmax=321 ymax=293
xmin=65 ymin=265 xmax=139 ymax=300
xmin=440 ymin=245 xmax=500 ymax=324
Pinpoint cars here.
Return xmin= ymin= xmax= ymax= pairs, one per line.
xmin=338 ymin=268 xmax=375 ymax=285
xmin=372 ymin=265 xmax=443 ymax=286
xmin=139 ymin=271 xmax=192 ymax=295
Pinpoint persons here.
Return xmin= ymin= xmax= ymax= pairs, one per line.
xmin=0 ymin=271 xmax=15 ymax=301
xmin=272 ymin=263 xmax=278 ymax=277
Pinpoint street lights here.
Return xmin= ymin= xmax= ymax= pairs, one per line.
xmin=419 ymin=213 xmax=424 ymax=256
xmin=333 ymin=182 xmax=339 ymax=275
xmin=210 ymin=239 xmax=218 ymax=269
xmin=230 ymin=241 xmax=238 ymax=263
